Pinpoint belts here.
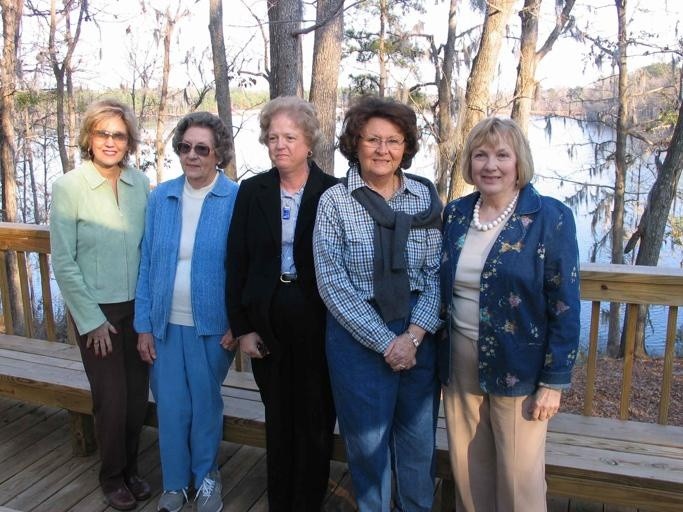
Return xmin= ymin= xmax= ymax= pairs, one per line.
xmin=280 ymin=272 xmax=297 ymax=284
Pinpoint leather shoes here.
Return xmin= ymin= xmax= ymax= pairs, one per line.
xmin=107 ymin=482 xmax=136 ymax=510
xmin=128 ymin=475 xmax=151 ymax=500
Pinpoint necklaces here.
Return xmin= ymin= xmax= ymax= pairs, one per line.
xmin=472 ymin=184 xmax=520 ymax=231
xmin=279 ymin=170 xmax=311 ymax=196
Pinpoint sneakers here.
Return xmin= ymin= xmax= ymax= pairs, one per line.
xmin=157 ymin=487 xmax=188 ymax=512
xmin=196 ymin=470 xmax=223 ymax=511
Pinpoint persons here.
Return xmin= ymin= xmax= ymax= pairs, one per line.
xmin=224 ymin=97 xmax=344 ymax=512
xmin=440 ymin=116 xmax=580 ymax=511
xmin=49 ymin=97 xmax=151 ymax=510
xmin=313 ymin=94 xmax=441 ymax=511
xmin=134 ymin=111 xmax=238 ymax=512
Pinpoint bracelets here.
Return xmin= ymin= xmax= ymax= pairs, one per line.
xmin=405 ymin=330 xmax=420 ymax=349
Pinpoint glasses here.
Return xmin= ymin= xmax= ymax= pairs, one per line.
xmin=94 ymin=130 xmax=128 ymax=141
xmin=177 ymin=142 xmax=209 ymax=156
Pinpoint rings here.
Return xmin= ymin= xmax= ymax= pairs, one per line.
xmin=93 ymin=340 xmax=100 ymax=345
xmin=399 ymin=364 xmax=406 ymax=371
